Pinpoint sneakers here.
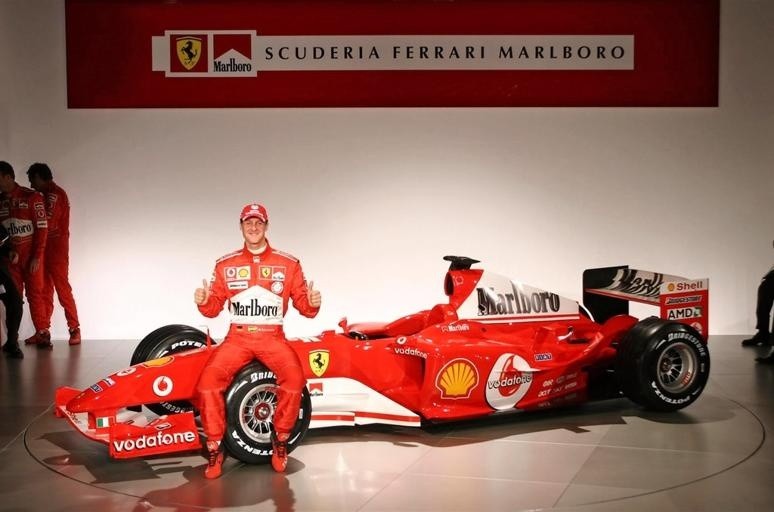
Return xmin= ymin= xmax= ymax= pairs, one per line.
xmin=204 ymin=439 xmax=224 ymax=480
xmin=270 ymin=431 xmax=289 ymax=472
xmin=754 ymin=350 xmax=773 ymax=363
xmin=2 ymin=342 xmax=24 ymax=359
xmin=67 ymin=327 xmax=82 ymax=345
xmin=24 ymin=331 xmax=53 ymax=345
xmin=741 ymin=330 xmax=769 ymax=346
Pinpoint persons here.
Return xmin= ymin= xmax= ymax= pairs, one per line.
xmin=26 ymin=162 xmax=82 ymax=350
xmin=741 ymin=268 xmax=774 ymax=364
xmin=0 ymin=222 xmax=25 ymax=359
xmin=0 ymin=160 xmax=52 ymax=345
xmin=194 ymin=203 xmax=321 ymax=479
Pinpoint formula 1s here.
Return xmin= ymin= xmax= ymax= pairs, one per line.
xmin=48 ymin=253 xmax=711 ymax=469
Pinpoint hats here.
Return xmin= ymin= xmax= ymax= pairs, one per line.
xmin=238 ymin=204 xmax=268 ymax=222
